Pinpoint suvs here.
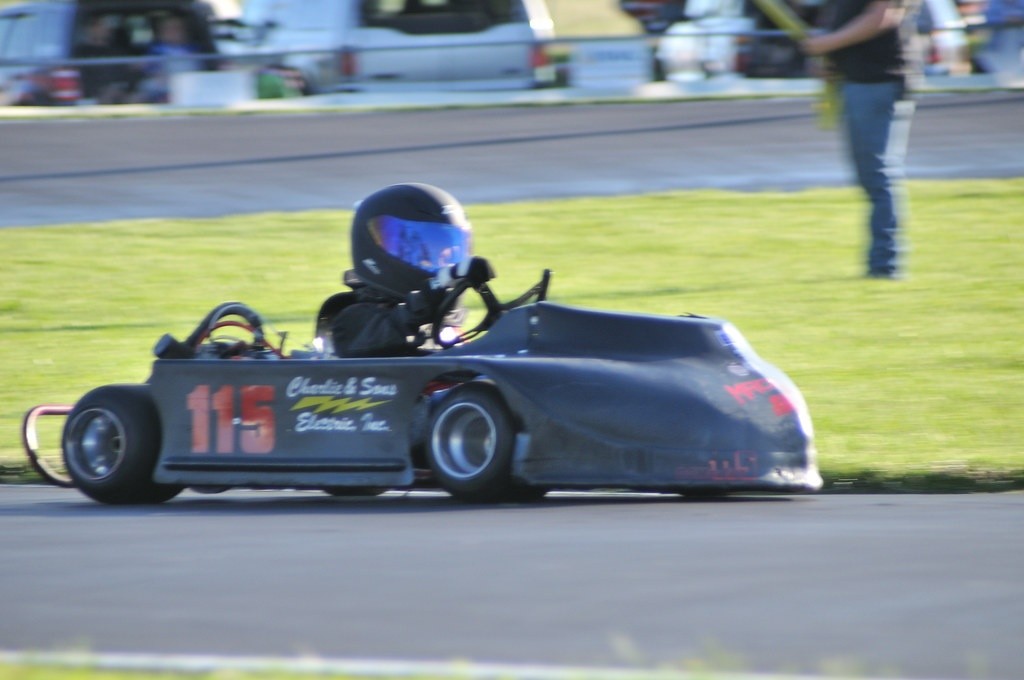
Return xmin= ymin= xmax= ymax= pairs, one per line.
xmin=621 ymin=0 xmax=965 ymax=89
xmin=0 ymin=0 xmax=224 ymax=106
xmin=232 ymin=0 xmax=557 ymax=99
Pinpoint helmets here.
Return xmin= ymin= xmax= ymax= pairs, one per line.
xmin=344 ymin=182 xmax=469 ymax=326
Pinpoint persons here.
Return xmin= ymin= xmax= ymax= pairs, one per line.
xmin=325 ymin=182 xmax=494 ymax=358
xmin=132 ymin=19 xmax=206 ymax=102
xmin=959 ymin=0 xmax=1002 ymax=75
xmin=804 ymin=0 xmax=923 ymax=278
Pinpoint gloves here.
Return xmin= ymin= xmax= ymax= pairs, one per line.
xmin=426 ymin=254 xmax=496 ymax=296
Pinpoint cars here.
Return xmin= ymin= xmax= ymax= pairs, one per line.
xmin=971 ymin=1 xmax=1024 ymax=78
